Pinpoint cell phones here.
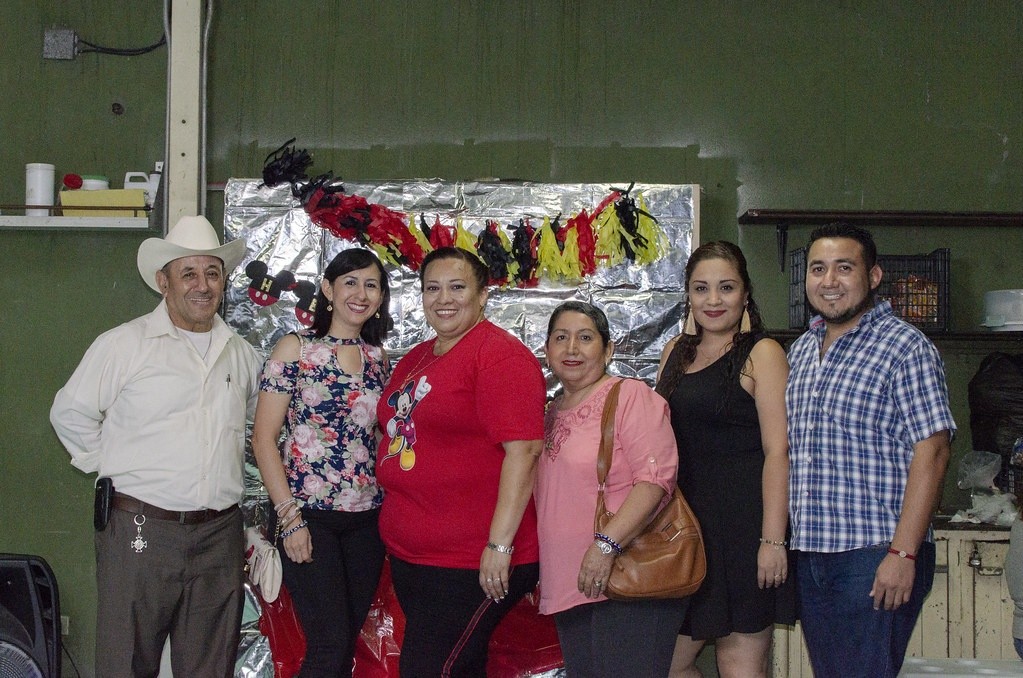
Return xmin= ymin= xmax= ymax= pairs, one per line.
xmin=93 ymin=478 xmax=112 ymax=533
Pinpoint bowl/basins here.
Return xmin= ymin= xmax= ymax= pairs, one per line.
xmin=981 ymin=288 xmax=1023 ymax=331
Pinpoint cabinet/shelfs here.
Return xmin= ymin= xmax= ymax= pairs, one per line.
xmin=737 ymin=209 xmax=1023 ymax=343
xmin=771 ymin=515 xmax=1023 ymax=678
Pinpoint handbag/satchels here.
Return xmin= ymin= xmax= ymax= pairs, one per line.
xmin=242 ymin=526 xmax=284 ymax=602
xmin=594 ymin=378 xmax=707 ymax=599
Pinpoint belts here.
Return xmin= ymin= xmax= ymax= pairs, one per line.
xmin=110 ymin=493 xmax=238 ymax=524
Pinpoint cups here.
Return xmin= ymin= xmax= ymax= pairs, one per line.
xmin=25 ymin=163 xmax=55 ymax=216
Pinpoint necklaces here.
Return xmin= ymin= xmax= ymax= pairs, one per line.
xmin=199 ymin=331 xmax=212 ymax=359
xmin=400 ymin=340 xmax=455 ymax=389
xmin=700 ymin=340 xmax=734 ymax=367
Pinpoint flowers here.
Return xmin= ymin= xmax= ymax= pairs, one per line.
xmin=56 ymin=174 xmax=83 ymax=205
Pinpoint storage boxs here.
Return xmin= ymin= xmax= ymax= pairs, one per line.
xmin=789 ymin=245 xmax=951 ymax=338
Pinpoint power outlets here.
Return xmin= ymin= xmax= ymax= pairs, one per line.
xmin=60 ymin=616 xmax=70 ymax=637
xmin=41 ymin=28 xmax=75 ymax=59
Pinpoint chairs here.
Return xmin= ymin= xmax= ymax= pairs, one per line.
xmin=0 ymin=553 xmax=61 ymax=678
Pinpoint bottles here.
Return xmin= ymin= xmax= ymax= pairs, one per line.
xmin=80 ymin=173 xmax=108 ymax=192
xmin=124 ymin=170 xmax=161 ymax=211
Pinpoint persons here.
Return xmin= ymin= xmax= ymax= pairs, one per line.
xmin=1004 ymin=502 xmax=1023 ymax=661
xmin=49 ymin=216 xmax=268 ymax=678
xmin=532 ymin=302 xmax=679 ymax=678
xmin=655 ymin=240 xmax=800 ymax=678
xmin=376 ymin=246 xmax=547 ymax=678
xmin=781 ymin=223 xmax=959 ymax=678
xmin=252 ymin=248 xmax=392 ymax=678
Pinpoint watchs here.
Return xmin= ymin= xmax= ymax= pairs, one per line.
xmin=594 ymin=539 xmax=617 ymax=556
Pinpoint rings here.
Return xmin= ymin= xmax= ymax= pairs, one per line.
xmin=594 ymin=583 xmax=601 ymax=587
xmin=775 ymin=574 xmax=781 ymax=577
xmin=494 ymin=577 xmax=499 ymax=582
xmin=487 ymin=578 xmax=491 ymax=582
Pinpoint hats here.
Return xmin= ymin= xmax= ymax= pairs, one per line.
xmin=136 ymin=215 xmax=246 ymax=294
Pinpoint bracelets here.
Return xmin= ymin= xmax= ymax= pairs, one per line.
xmin=487 ymin=542 xmax=514 ymax=555
xmin=888 ymin=549 xmax=917 ymax=560
xmin=275 ymin=498 xmax=307 ymax=538
xmin=594 ymin=532 xmax=624 ymax=555
xmin=760 ymin=539 xmax=787 ymax=547
